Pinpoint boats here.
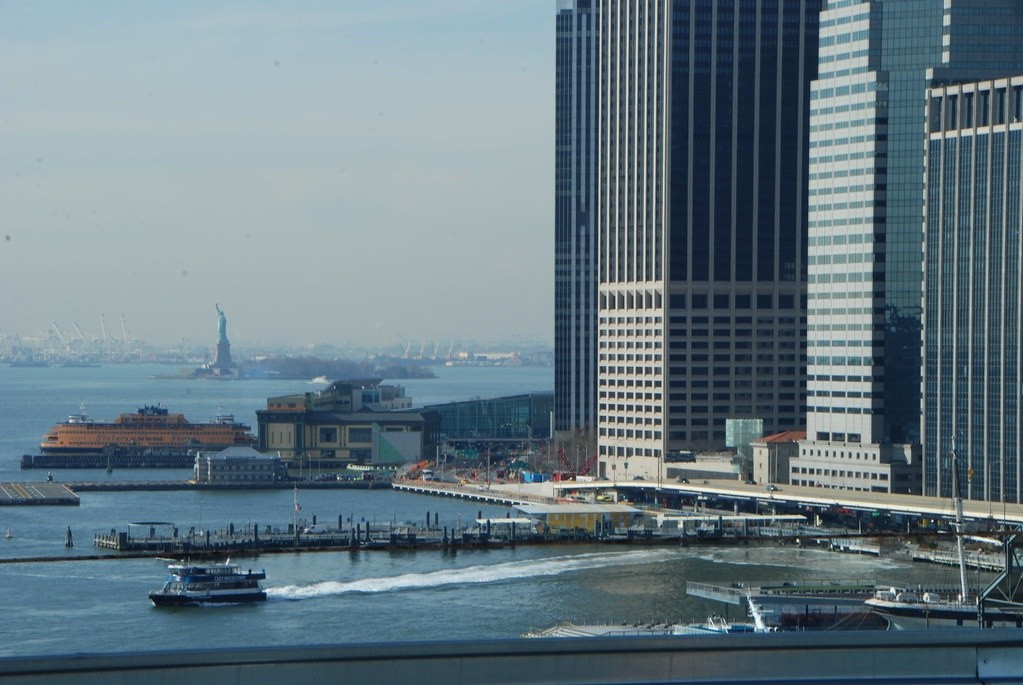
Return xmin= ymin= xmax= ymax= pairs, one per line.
xmin=148 ymin=552 xmax=267 ymax=606
xmin=312 ymin=374 xmax=327 ymax=384
xmin=39 ymin=403 xmax=256 ymax=453
xmin=516 ymin=434 xmax=1022 ymax=638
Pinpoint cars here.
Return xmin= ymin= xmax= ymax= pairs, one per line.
xmin=633 ymin=476 xmax=644 ymax=480
xmin=745 ymin=480 xmax=757 ymax=485
xmin=768 ymin=485 xmax=777 ymax=491
xmin=677 ymin=478 xmax=689 ymax=484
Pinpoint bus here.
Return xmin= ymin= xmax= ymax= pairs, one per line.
xmin=422 ymin=469 xmax=433 ymax=481
